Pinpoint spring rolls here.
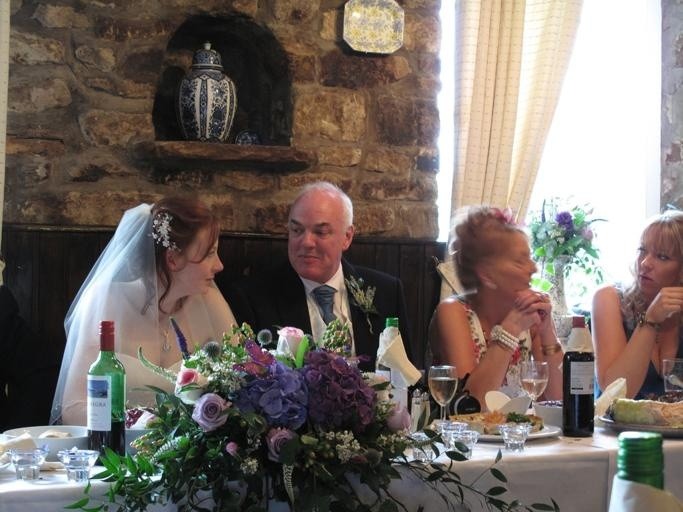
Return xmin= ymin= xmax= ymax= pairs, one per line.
xmin=608 ymin=399 xmax=683 ymax=426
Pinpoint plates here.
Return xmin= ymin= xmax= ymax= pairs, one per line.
xmin=431 ymin=419 xmax=561 ymax=443
xmin=599 ymin=414 xmax=683 ymax=438
xmin=340 ymin=0 xmax=405 ymax=55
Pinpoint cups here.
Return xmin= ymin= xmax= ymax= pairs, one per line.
xmin=497 ymin=424 xmax=533 ymax=453
xmin=6 ymin=448 xmax=48 ymax=480
xmin=57 ymin=450 xmax=101 ymax=482
xmin=663 ymin=357 xmax=683 ymax=392
xmin=436 ymin=422 xmax=480 ymax=459
xmin=411 ymin=432 xmax=433 ymax=462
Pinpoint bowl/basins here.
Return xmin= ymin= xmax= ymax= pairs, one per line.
xmin=2 ymin=423 xmax=88 ymax=462
xmin=532 ymin=400 xmax=563 ymax=429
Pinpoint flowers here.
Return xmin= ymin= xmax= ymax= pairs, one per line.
xmin=150 ymin=214 xmax=179 ymax=251
xmin=100 ymin=325 xmax=530 ymax=511
xmin=528 ymin=202 xmax=606 ymax=267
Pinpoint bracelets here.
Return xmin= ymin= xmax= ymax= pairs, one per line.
xmin=638 ymin=311 xmax=660 ymax=329
xmin=541 ymin=342 xmax=561 ymax=355
xmin=488 ymin=324 xmax=520 ymax=350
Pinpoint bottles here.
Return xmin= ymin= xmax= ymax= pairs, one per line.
xmin=562 ymin=315 xmax=595 ymax=436
xmin=86 ymin=320 xmax=126 ymax=467
xmin=375 ymin=316 xmax=410 ymax=417
xmin=409 ymin=388 xmax=431 ymax=432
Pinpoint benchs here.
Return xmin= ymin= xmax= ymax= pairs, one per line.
xmin=0 ymin=223 xmax=450 ymax=422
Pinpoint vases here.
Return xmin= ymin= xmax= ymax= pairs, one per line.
xmin=539 ymin=253 xmax=587 ymax=334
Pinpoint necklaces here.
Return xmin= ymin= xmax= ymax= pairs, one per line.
xmin=147 ymin=305 xmax=177 ymax=351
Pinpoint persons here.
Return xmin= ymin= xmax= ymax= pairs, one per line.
xmin=0 ymin=286 xmax=47 ymax=432
xmin=424 ymin=207 xmax=564 ymax=411
xmin=49 ymin=194 xmax=242 ymax=427
xmin=228 ymin=180 xmax=422 ymax=415
xmin=593 ymin=216 xmax=683 ymax=400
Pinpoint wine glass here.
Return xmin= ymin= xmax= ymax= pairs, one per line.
xmin=427 ymin=366 xmax=458 ymax=426
xmin=520 ymin=360 xmax=548 ymax=416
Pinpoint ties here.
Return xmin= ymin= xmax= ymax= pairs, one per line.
xmin=313 ymin=285 xmax=337 ymax=328
